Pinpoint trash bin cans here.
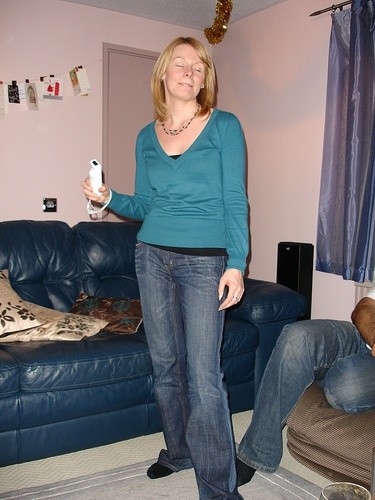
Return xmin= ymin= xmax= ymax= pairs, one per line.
xmin=277 ymin=240 xmax=313 ymax=320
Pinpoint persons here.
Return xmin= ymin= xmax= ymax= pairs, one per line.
xmin=237 ymin=284 xmax=375 ymax=487
xmin=26 ymin=86 xmax=37 ymax=103
xmin=82 ymin=36 xmax=249 ymax=500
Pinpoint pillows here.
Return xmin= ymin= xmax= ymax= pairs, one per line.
xmin=0 ymin=298 xmax=111 ymax=342
xmin=68 ymin=289 xmax=144 ymax=335
xmin=0 ymin=268 xmax=47 ymax=338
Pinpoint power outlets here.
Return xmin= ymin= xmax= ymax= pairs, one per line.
xmin=42 ymin=197 xmax=57 ymax=213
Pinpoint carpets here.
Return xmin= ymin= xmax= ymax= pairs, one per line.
xmin=0 ymin=440 xmax=323 ymax=500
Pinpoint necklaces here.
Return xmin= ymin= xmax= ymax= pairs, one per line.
xmin=162 ymin=106 xmax=200 ymax=137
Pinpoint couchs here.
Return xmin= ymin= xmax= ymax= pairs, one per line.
xmin=0 ymin=220 xmax=309 ymax=470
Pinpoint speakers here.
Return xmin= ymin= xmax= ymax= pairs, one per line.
xmin=276 ymin=242 xmax=314 ymax=322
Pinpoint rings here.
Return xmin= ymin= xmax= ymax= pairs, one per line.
xmin=233 ymin=295 xmax=238 ymax=300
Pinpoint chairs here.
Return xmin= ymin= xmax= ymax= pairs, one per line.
xmin=287 ymin=380 xmax=375 ymax=500
